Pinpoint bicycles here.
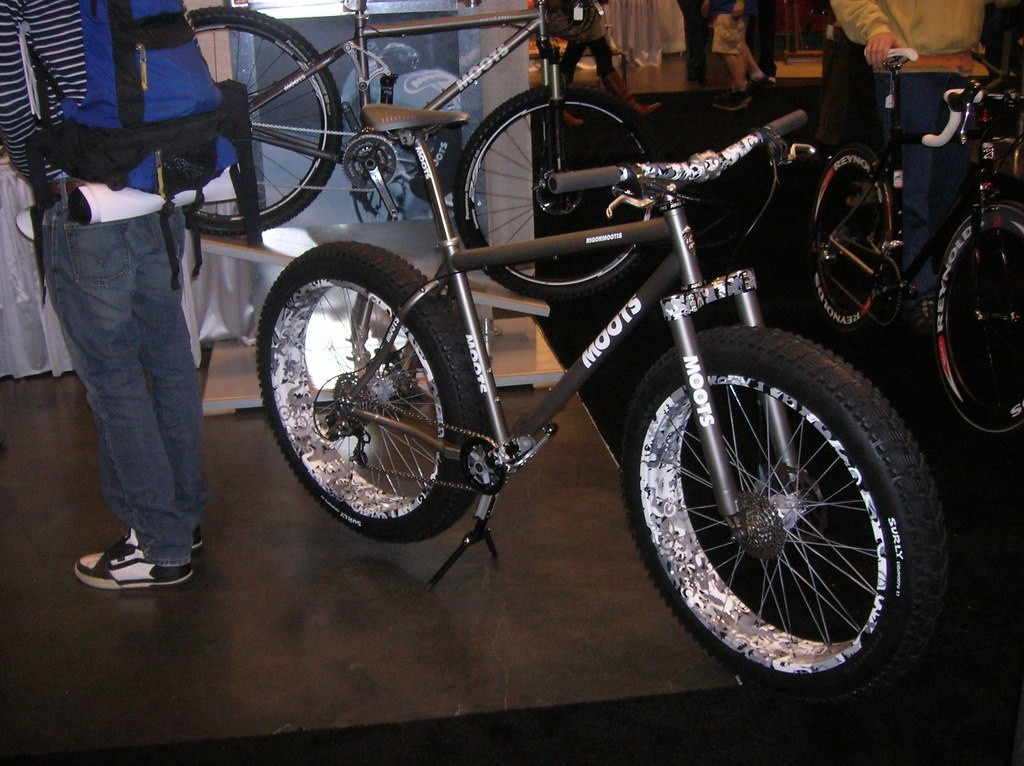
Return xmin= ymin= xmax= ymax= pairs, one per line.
xmin=154 ymin=0 xmax=665 ymax=304
xmin=806 ymin=44 xmax=1024 ymax=447
xmin=255 ymin=108 xmax=953 ymax=708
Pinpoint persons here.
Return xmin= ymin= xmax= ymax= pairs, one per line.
xmin=0 ymin=0 xmax=212 ymax=590
xmin=674 ymin=0 xmax=777 ymax=112
xmin=544 ymin=0 xmax=663 ymax=128
xmin=831 ymin=0 xmax=1022 ymax=345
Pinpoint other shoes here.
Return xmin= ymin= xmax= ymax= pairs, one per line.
xmin=901 ymin=297 xmax=938 ymax=336
xmin=748 ymin=74 xmax=777 ymax=97
xmin=712 ymin=87 xmax=752 ymax=112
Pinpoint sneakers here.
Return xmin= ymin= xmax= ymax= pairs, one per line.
xmin=74 ymin=523 xmax=203 ymax=590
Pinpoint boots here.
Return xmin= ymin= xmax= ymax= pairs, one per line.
xmin=559 ymin=67 xmax=585 ymax=125
xmin=601 ymin=69 xmax=663 ymax=119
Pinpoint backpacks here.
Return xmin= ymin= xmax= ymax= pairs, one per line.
xmin=24 ymin=0 xmax=241 ymax=217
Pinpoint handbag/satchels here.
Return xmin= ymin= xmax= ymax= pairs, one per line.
xmin=542 ymin=0 xmax=599 ymax=44
